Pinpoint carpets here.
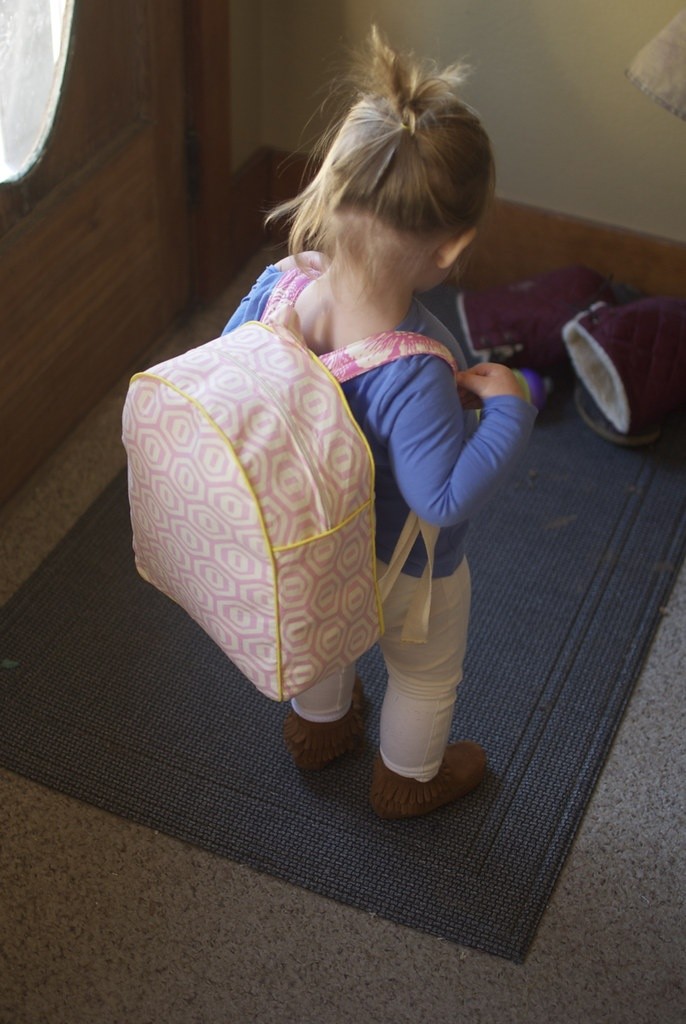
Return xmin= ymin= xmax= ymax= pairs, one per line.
xmin=0 ymin=282 xmax=686 ymax=965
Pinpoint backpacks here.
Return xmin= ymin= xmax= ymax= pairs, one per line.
xmin=121 ymin=261 xmax=460 ymax=703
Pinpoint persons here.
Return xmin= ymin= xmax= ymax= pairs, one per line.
xmin=220 ymin=24 xmax=539 ymax=816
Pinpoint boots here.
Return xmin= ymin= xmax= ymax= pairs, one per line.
xmin=458 ymin=266 xmax=634 ymax=376
xmin=368 ymin=741 xmax=485 ymax=820
xmin=562 ymin=293 xmax=686 ymax=446
xmin=284 ymin=672 xmax=363 ymax=771
